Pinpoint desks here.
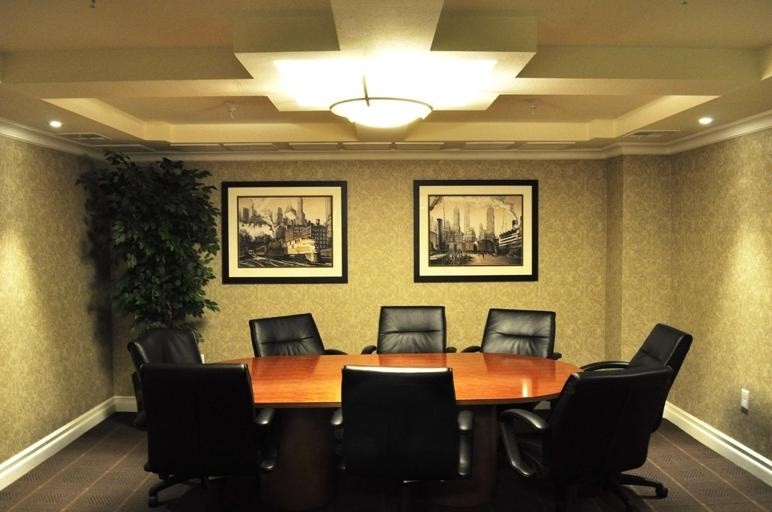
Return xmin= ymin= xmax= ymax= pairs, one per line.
xmin=212 ymin=353 xmax=584 ymax=408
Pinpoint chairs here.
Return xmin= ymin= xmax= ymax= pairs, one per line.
xmin=249 ymin=312 xmax=348 ymax=358
xmin=461 ymin=308 xmax=562 ymax=360
xmin=499 ymin=368 xmax=672 ymax=509
xmin=127 ymin=325 xmax=203 ymax=496
xmin=579 ymin=322 xmax=694 ymax=499
xmin=330 ymin=365 xmax=474 ymax=510
xmin=360 ymin=305 xmax=457 ymax=354
xmin=132 ymin=364 xmax=293 ymax=508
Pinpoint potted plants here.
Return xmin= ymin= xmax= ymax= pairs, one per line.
xmin=71 ymin=142 xmax=226 ymax=431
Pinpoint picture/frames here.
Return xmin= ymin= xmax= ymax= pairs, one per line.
xmin=412 ymin=175 xmax=539 ymax=284
xmin=217 ymin=177 xmax=349 ymax=286
